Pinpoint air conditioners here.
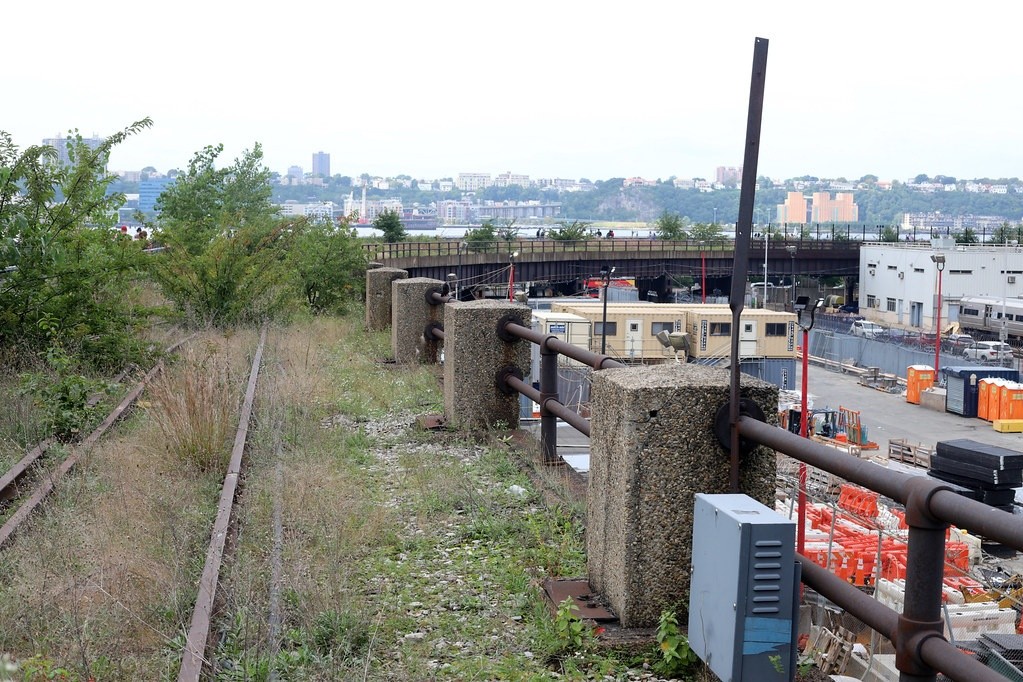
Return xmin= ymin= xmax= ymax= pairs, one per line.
xmin=1008 ymin=277 xmax=1015 ymax=283
xmin=898 ymin=272 xmax=904 ymax=279
xmin=873 ymin=299 xmax=880 ymax=305
xmin=869 ymin=269 xmax=875 ymax=274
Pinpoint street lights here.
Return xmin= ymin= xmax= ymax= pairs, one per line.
xmin=929 ymin=254 xmax=946 ymax=382
xmin=786 ymin=245 xmax=798 ymax=313
xmin=600 ymin=265 xmax=616 ymax=355
xmin=508 ymin=250 xmax=519 ymax=302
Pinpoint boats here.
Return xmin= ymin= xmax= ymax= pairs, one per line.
xmin=791 ymin=294 xmax=824 ymax=602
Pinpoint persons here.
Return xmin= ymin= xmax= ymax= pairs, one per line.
xmin=607 ymin=231 xmax=614 ymax=238
xmin=537 ymin=228 xmax=545 ymax=238
xmin=121 ymin=225 xmax=132 ymax=240
xmin=134 ymin=228 xmax=142 ymax=239
xmin=596 ymin=229 xmax=602 ymax=237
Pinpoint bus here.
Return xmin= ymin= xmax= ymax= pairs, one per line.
xmin=751 ymin=282 xmax=774 ymax=290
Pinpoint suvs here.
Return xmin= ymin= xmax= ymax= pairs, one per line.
xmin=916 ymin=332 xmax=943 ymax=351
xmin=962 ymin=341 xmax=1014 ymax=367
xmin=946 ymin=333 xmax=975 ymax=356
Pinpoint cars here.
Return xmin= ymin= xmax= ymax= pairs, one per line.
xmin=849 ymin=320 xmax=884 ymax=340
xmin=879 ymin=328 xmax=923 ymax=347
